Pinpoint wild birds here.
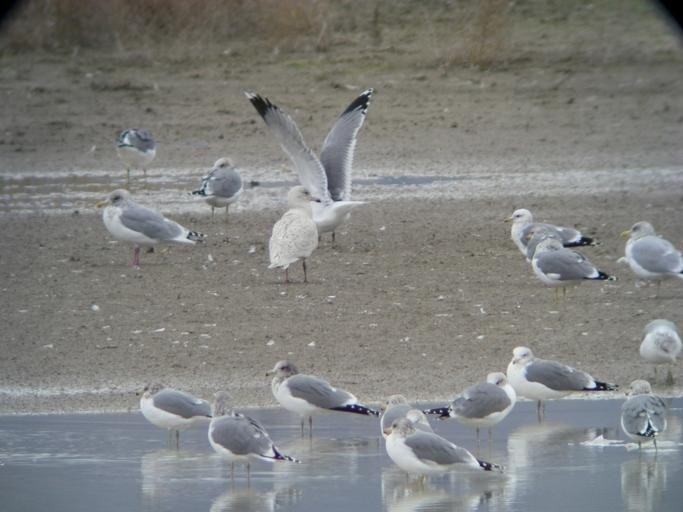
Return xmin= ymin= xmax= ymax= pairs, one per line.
xmin=243 ymin=87 xmax=374 ymax=285
xmin=507 ymin=346 xmax=619 ymax=426
xmin=620 ymin=379 xmax=668 ymax=452
xmin=116 ymin=127 xmax=156 ymax=185
xmin=381 ymin=393 xmax=506 ymax=487
xmin=264 ymin=359 xmax=380 ymax=439
xmin=638 ymin=318 xmax=683 ymax=386
xmin=186 ymin=157 xmax=244 ymax=225
xmin=135 ymin=379 xmax=212 ymax=454
xmin=423 ymin=372 xmax=515 ymax=460
xmin=96 ymin=188 xmax=209 ymax=268
xmin=503 ymin=207 xmax=683 ymax=300
xmin=207 ymin=389 xmax=302 ymax=486
